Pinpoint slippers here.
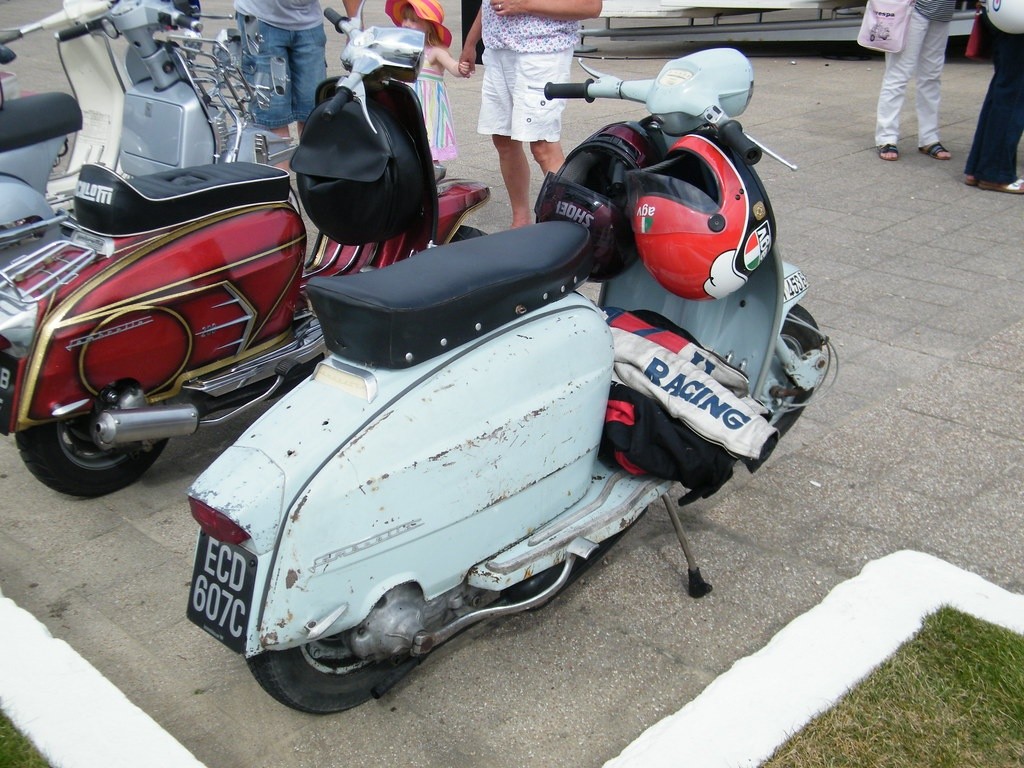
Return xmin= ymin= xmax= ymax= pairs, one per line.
xmin=978 ymin=179 xmax=1024 ymax=195
xmin=964 ymin=179 xmax=977 ymax=185
xmin=919 ymin=141 xmax=950 ymax=160
xmin=879 ymin=144 xmax=898 ymax=160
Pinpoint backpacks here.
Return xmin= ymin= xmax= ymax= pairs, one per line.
xmin=289 ymin=95 xmax=422 ymax=243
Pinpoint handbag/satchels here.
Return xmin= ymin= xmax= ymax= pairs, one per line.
xmin=965 ymin=11 xmax=991 ymax=61
xmin=857 ymin=0 xmax=914 ymax=53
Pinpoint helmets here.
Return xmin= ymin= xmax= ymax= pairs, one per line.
xmin=632 ymin=132 xmax=775 ymax=301
xmin=532 ymin=123 xmax=650 ymax=280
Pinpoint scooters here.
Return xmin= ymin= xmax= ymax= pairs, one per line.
xmin=0 ymin=0 xmax=493 ymax=499
xmin=181 ymin=48 xmax=839 ymax=716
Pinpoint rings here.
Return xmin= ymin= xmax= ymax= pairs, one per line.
xmin=499 ymin=4 xmax=504 ymax=10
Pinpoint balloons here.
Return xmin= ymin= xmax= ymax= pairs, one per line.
xmin=985 ymin=0 xmax=1024 ymax=34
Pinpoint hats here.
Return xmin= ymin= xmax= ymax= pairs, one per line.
xmin=384 ymin=0 xmax=452 ymax=49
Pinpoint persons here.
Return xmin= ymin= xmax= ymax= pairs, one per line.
xmin=875 ymin=0 xmax=1024 ymax=194
xmin=384 ymin=0 xmax=603 ymax=230
xmin=233 ymin=0 xmax=364 ymax=173
